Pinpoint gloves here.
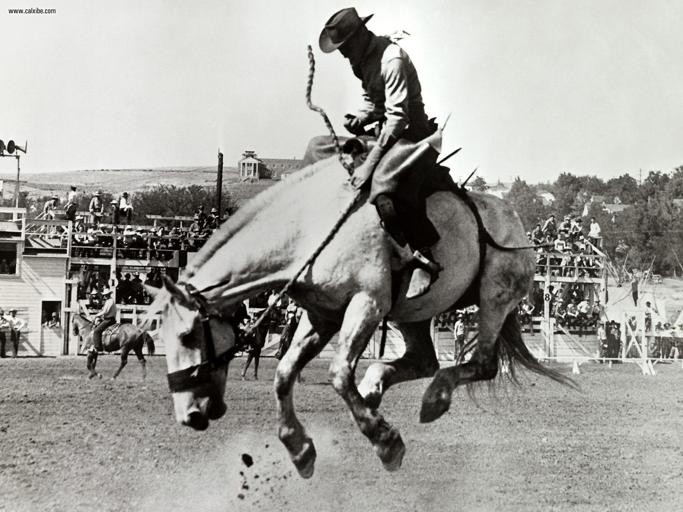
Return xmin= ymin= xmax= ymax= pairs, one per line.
xmin=347 ymin=145 xmax=384 ymax=190
xmin=343 ymin=114 xmax=364 ymax=134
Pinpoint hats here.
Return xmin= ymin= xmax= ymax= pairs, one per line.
xmin=318 ymin=7 xmax=374 ymax=53
xmin=100 ymin=288 xmax=113 ymax=296
xmin=8 ymin=309 xmax=17 ymax=313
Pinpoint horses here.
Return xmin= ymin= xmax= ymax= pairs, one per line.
xmin=142 ymin=152 xmax=581 ymax=479
xmin=72 ymin=313 xmax=155 ymax=381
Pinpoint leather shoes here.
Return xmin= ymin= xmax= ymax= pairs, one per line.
xmin=405 ymin=268 xmax=433 ymax=299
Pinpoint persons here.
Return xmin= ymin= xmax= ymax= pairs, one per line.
xmin=40 ymin=185 xmax=302 ymax=382
xmin=434 ymin=215 xmax=682 ymax=361
xmin=0 ymin=307 xmax=9 ymax=358
xmin=317 ymin=7 xmax=442 ymax=300
xmin=8 ymin=309 xmax=25 ymax=359
